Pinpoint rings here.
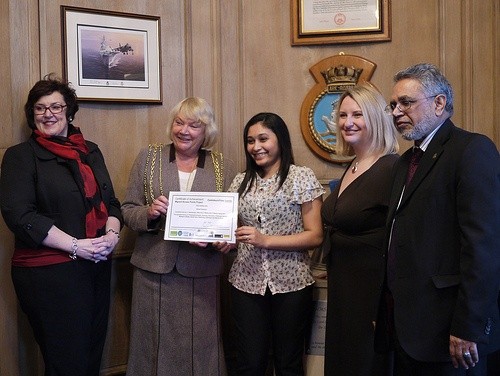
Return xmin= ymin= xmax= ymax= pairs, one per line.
xmin=464 ymin=352 xmax=470 ymax=358
xmin=246 ymin=236 xmax=249 ymax=240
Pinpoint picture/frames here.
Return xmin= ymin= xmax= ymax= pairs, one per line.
xmin=60 ymin=4 xmax=164 ymax=106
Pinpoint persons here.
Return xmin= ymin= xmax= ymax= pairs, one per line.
xmin=213 ymin=113 xmax=326 ymax=376
xmin=0 ymin=74 xmax=125 ymax=376
xmin=384 ymin=63 xmax=500 ymax=376
xmin=320 ymin=81 xmax=401 ymax=376
xmin=120 ymin=96 xmax=231 ymax=376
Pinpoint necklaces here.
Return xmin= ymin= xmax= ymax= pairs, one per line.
xmin=351 ymin=159 xmax=363 ymax=174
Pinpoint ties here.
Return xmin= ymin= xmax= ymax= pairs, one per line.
xmin=405 ymin=148 xmax=423 ymax=188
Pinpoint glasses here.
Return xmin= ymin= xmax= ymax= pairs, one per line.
xmin=384 ymin=95 xmax=438 ymax=113
xmin=32 ymin=103 xmax=67 ymax=115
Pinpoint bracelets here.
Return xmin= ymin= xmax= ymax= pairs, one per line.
xmin=69 ymin=238 xmax=78 ymax=260
xmin=107 ymin=228 xmax=120 ymax=240
